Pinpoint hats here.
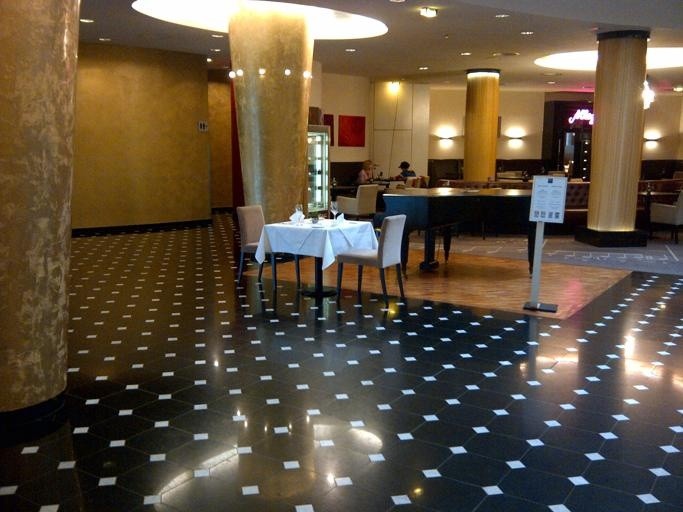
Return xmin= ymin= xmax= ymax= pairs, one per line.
xmin=398 ymin=161 xmax=410 ymax=168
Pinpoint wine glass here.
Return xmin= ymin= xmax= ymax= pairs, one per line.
xmin=329 ymin=201 xmax=338 ymax=222
xmin=295 ymin=204 xmax=303 ymax=223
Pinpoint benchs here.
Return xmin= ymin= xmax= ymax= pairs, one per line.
xmin=546 ymin=181 xmax=588 ymax=235
xmin=636 ymin=180 xmax=682 ymax=215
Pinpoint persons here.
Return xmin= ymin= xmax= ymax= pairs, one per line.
xmin=380 ymin=160 xmax=416 ymax=182
xmin=355 ymin=159 xmax=373 ymax=184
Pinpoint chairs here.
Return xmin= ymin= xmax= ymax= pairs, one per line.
xmin=337 ymin=214 xmax=407 ymax=301
xmin=235 ymin=205 xmax=299 ymax=291
xmin=650 ymin=189 xmax=682 ymax=244
xmin=335 ymin=175 xmax=430 ymax=222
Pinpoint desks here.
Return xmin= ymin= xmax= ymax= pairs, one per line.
xmin=638 ymin=191 xmax=677 ymax=235
